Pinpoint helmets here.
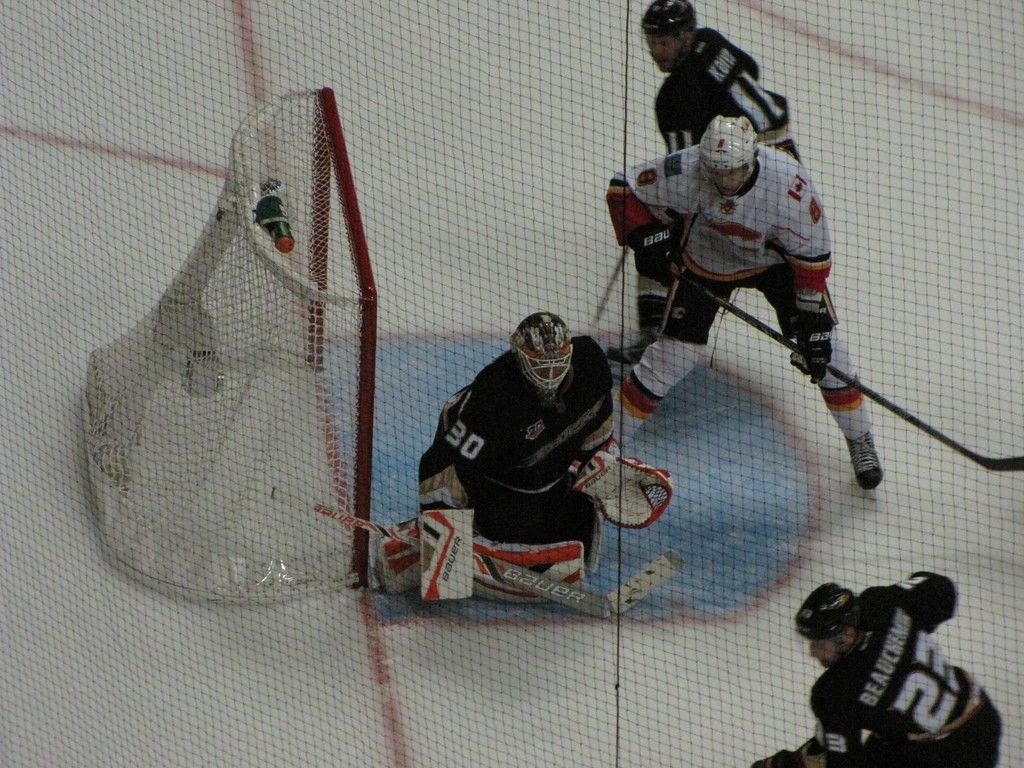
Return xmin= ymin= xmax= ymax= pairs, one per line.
xmin=794 ymin=582 xmax=861 ymax=640
xmin=513 ymin=311 xmax=573 ymax=394
xmin=699 ymin=115 xmax=756 ymax=196
xmin=640 ymin=0 xmax=697 ymax=73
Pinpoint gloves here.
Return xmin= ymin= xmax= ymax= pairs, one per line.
xmin=790 ymin=309 xmax=834 ymax=384
xmin=626 ymin=221 xmax=682 ymax=287
xmin=749 ymin=748 xmax=806 ymax=768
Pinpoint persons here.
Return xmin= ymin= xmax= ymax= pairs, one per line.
xmin=641 ymin=1 xmax=801 ymax=168
xmin=745 ymin=569 xmax=1005 ymax=768
xmin=604 ymin=114 xmax=881 ymax=501
xmin=375 ymin=313 xmax=613 ymax=591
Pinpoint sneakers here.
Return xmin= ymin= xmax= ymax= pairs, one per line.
xmin=846 ymin=432 xmax=883 ymax=489
xmin=608 ymin=334 xmax=662 ymax=381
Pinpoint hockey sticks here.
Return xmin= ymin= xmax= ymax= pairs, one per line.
xmin=587 ymin=241 xmax=632 ymax=328
xmin=314 ymin=501 xmax=687 ymax=622
xmin=670 ymin=263 xmax=1024 ymax=473
